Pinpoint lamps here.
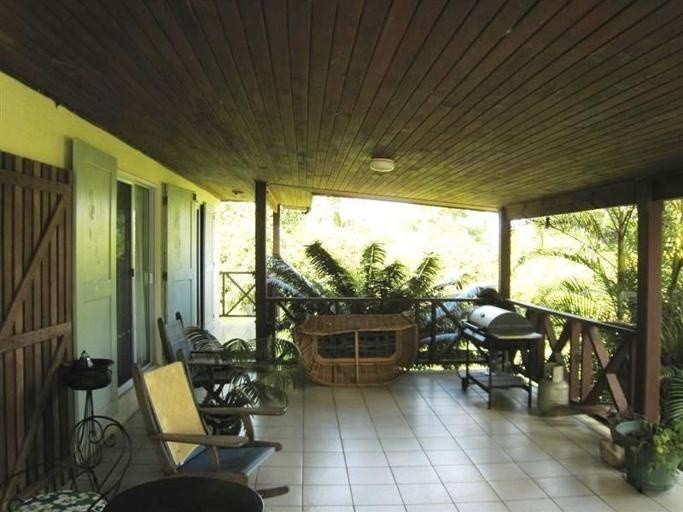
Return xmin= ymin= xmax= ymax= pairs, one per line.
xmin=368 ymin=158 xmax=396 ymax=172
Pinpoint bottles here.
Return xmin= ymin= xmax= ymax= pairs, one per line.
xmin=79 ymin=351 xmax=95 ymax=369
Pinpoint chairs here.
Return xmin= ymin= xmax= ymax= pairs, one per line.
xmin=0 ymin=414 xmax=131 ymax=512
xmin=155 ymin=312 xmax=242 ymax=406
xmin=130 ymin=348 xmax=290 ymax=498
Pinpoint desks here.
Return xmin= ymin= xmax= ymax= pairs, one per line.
xmin=457 ymin=319 xmax=544 ymax=409
xmin=188 ymin=364 xmax=240 ymax=437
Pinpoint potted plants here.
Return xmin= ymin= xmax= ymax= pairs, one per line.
xmin=61 ymin=350 xmax=114 ymax=491
xmin=184 ymin=326 xmax=315 ymax=436
xmin=614 ymin=418 xmax=683 ymax=493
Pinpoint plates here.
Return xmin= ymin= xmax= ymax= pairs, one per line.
xmin=62 ymin=359 xmax=116 ymax=373
